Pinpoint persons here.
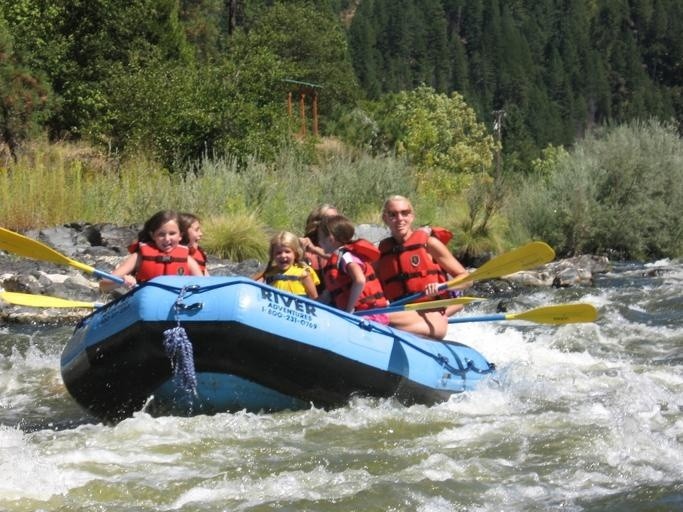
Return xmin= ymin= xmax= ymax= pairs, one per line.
xmin=257 ymin=195 xmax=474 ymax=340
xmin=100 ymin=210 xmax=210 ymax=290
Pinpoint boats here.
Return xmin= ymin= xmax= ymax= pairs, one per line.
xmin=60 ymin=276 xmax=495 ymax=415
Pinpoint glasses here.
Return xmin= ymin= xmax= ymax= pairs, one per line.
xmin=386 ymin=209 xmax=412 ymax=217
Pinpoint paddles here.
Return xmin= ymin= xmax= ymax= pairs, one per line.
xmin=1 ymin=291 xmax=106 ymax=309
xmin=387 ymin=242 xmax=556 ymax=305
xmin=354 ymin=298 xmax=488 ymax=315
xmin=449 ymin=303 xmax=597 ymax=324
xmin=0 ymin=228 xmax=140 ymax=287
